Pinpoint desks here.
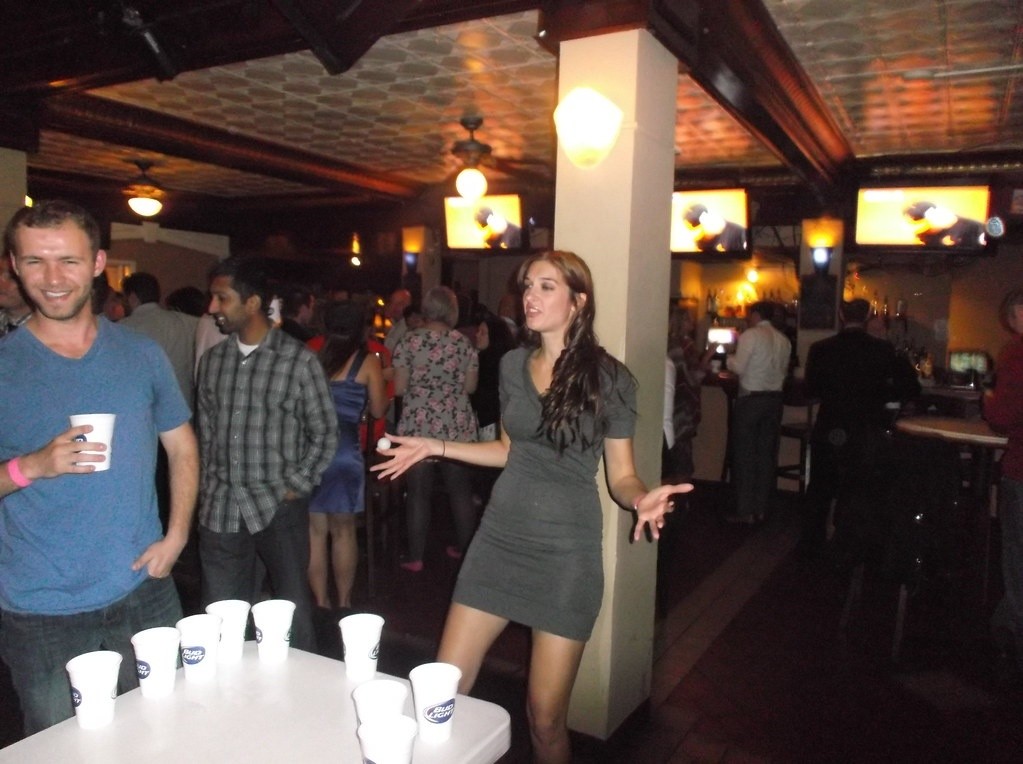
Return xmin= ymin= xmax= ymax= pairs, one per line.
xmin=899 ymin=417 xmax=1009 ymax=625
xmin=0 ymin=641 xmax=510 ymax=764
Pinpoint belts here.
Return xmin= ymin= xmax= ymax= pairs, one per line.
xmin=741 ymin=391 xmax=782 ymax=399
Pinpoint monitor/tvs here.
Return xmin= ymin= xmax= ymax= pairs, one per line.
xmin=852 ymin=181 xmax=1001 ymax=254
xmin=670 ymin=184 xmax=753 ymax=261
xmin=440 ymin=190 xmax=529 ymax=256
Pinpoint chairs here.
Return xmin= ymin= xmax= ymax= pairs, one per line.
xmin=775 ymin=388 xmax=823 ymax=494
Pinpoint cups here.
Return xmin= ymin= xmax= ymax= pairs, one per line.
xmin=267 ymin=299 xmax=283 ymax=325
xmin=69 ymin=414 xmax=117 ymax=472
xmin=65 ymin=650 xmax=124 ymax=730
xmin=351 ymin=679 xmax=409 ymax=724
xmin=251 ymin=599 xmax=297 ymax=663
xmin=175 ymin=614 xmax=224 ymax=684
xmin=357 ymin=714 xmax=419 ymax=764
xmin=408 ymin=662 xmax=463 ymax=741
xmin=339 ymin=613 xmax=386 ymax=683
xmin=130 ymin=627 xmax=181 ymax=707
xmin=205 ymin=600 xmax=252 ymax=665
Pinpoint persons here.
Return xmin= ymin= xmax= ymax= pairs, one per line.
xmin=669 ymin=304 xmax=718 ymax=512
xmin=0 ymin=226 xmax=542 ymax=523
xmin=0 ymin=201 xmax=200 ymax=736
xmin=797 ymin=298 xmax=927 ymax=572
xmin=196 ymin=252 xmax=341 ymax=654
xmin=717 ymin=302 xmax=791 ymax=527
xmin=392 ymin=286 xmax=479 ymax=572
xmin=110 ymin=271 xmax=201 ymax=430
xmin=983 ymin=288 xmax=1022 ymax=708
xmin=369 ymin=250 xmax=694 ymax=764
xmin=307 ymin=302 xmax=394 ymax=624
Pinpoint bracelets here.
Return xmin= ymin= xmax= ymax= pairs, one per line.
xmin=8 ymin=456 xmax=33 ymax=487
xmin=634 ymin=495 xmax=647 ymax=510
xmin=441 ymin=439 xmax=445 ymax=458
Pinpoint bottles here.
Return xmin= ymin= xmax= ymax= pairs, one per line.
xmin=705 ymin=287 xmax=799 ymax=320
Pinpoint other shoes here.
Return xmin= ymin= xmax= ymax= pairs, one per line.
xmin=394 ymin=554 xmax=424 ymax=572
xmin=334 ymin=605 xmax=355 ymax=626
xmin=312 ymin=608 xmax=337 ymax=631
xmin=719 ymin=508 xmax=768 ymax=524
xmin=969 ymin=637 xmax=1023 ymax=664
xmin=447 ymin=546 xmax=467 ymax=559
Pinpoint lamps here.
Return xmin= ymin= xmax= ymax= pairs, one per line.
xmin=128 ymin=163 xmax=162 ymax=216
xmin=553 ymin=90 xmax=624 ymax=166
xmin=452 ymin=119 xmax=491 ymax=199
